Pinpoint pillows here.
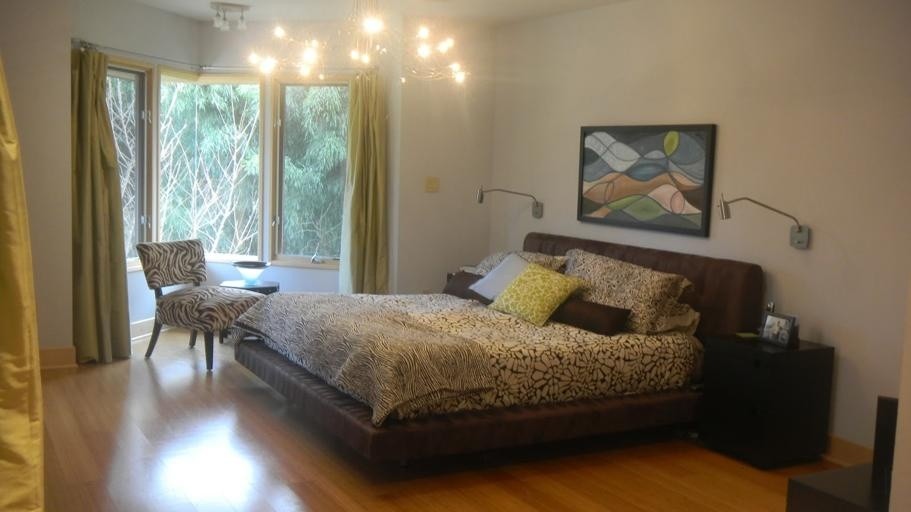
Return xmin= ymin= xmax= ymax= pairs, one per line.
xmin=563 ymin=249 xmax=691 ymax=333
xmin=469 ymin=252 xmax=529 ymax=300
xmin=551 ymin=297 xmax=631 ymax=336
xmin=478 ymin=250 xmax=567 ymax=279
xmin=488 ymin=261 xmax=587 ymax=328
xmin=443 ymin=272 xmax=493 ymax=305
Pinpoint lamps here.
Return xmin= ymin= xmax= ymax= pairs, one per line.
xmin=245 ymin=0 xmax=471 ymax=87
xmin=718 ymin=194 xmax=810 ymax=250
xmin=475 ymin=186 xmax=543 ymax=219
xmin=208 ymin=2 xmax=251 ymax=33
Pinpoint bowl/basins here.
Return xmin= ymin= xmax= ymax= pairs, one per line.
xmin=233 ymin=262 xmax=272 ymax=286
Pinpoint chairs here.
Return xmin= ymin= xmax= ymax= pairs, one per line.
xmin=137 ymin=239 xmax=268 ymax=370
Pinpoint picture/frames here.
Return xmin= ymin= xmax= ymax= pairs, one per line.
xmin=577 ymin=125 xmax=715 ymax=238
xmin=760 ymin=312 xmax=795 ymax=348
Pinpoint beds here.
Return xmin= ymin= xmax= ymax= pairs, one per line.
xmin=234 ymin=232 xmax=765 ymax=473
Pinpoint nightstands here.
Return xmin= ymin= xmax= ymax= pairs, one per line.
xmin=701 ymin=333 xmax=834 ymax=469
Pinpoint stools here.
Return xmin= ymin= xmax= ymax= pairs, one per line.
xmin=218 ymin=279 xmax=280 ymax=344
